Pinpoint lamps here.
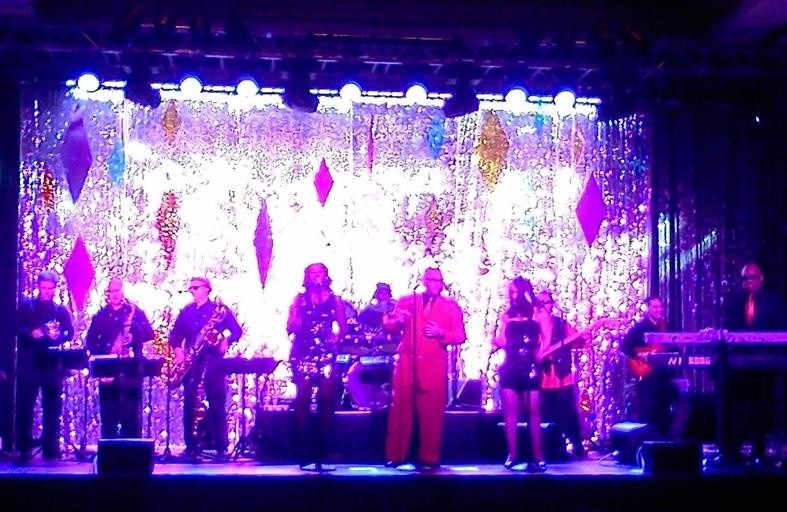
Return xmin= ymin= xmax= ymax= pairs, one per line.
xmin=441 ymin=77 xmax=480 ymax=119
xmin=120 ymin=72 xmax=163 ymax=110
xmin=277 ymin=77 xmax=321 ymax=114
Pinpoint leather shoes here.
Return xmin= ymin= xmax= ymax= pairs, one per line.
xmin=504 ymin=457 xmax=548 ymax=472
xmin=384 ymin=460 xmax=441 ymax=471
xmin=180 ymin=449 xmax=230 ymax=462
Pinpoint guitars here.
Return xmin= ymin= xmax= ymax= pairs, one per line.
xmin=629 ymin=344 xmax=667 ymax=380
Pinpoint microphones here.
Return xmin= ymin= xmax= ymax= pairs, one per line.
xmin=524 ymin=290 xmax=535 ymax=306
xmin=315 ymin=277 xmax=322 ymax=287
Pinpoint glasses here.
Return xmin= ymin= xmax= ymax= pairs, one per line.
xmin=188 ymin=284 xmax=207 ymax=290
xmin=535 ymin=299 xmax=553 ymax=304
xmin=742 ymin=274 xmax=762 ymax=282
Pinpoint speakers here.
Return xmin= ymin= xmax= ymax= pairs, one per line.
xmin=447 ymin=379 xmax=483 ymax=409
xmin=643 ymin=442 xmax=703 ymax=477
xmin=496 ymin=422 xmax=561 ymax=460
xmin=97 ymin=439 xmax=155 ymax=478
xmin=610 ymin=422 xmax=665 ymax=465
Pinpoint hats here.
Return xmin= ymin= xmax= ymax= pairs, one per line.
xmin=419 ymin=267 xmax=444 ymax=280
xmin=191 ymin=276 xmax=213 ymax=293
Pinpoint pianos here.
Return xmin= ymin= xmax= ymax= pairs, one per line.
xmin=644 ymin=329 xmax=786 ymax=373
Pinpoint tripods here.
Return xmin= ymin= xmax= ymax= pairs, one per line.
xmin=447 ymin=343 xmax=486 ymax=413
xmin=86 ymin=372 xmax=131 ymax=463
xmin=228 ymin=373 xmax=260 ymax=464
xmin=22 ymin=359 xmax=86 ymax=462
xmin=558 ymin=315 xmax=583 ymax=466
xmin=153 ymin=327 xmax=202 ymax=463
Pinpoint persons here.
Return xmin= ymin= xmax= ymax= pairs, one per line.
xmin=10 ymin=270 xmax=75 ymax=462
xmin=358 ymin=280 xmax=396 ymax=351
xmin=167 ymin=276 xmax=242 ymax=464
xmin=84 ymin=277 xmax=156 ymax=440
xmin=728 ymin=261 xmax=778 ymax=462
xmin=533 ymin=288 xmax=586 ymax=458
xmin=286 ymin=263 xmax=347 ymax=467
xmin=620 ymin=296 xmax=677 ymax=428
xmin=380 ymin=269 xmax=467 ymax=465
xmin=492 ymin=276 xmax=551 ymax=471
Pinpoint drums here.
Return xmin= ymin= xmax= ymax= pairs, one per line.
xmin=340 ymin=321 xmax=370 ymax=356
xmin=345 ymin=359 xmax=393 ymax=409
xmin=372 ymin=322 xmax=402 ymax=354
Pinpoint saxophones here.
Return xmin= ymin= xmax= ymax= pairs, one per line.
xmin=161 ymin=298 xmax=226 ymax=388
xmin=101 ymin=299 xmax=136 ymax=383
